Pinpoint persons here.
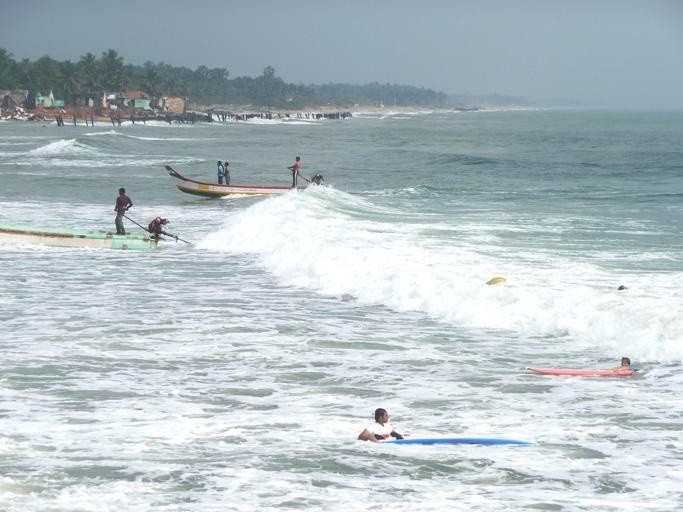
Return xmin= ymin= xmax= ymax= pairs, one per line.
xmin=217 ymin=160 xmax=225 ymax=185
xmin=357 ymin=408 xmax=405 ymax=441
xmin=310 ymin=173 xmax=324 ymax=185
xmin=223 ymin=162 xmax=230 ymax=185
xmin=287 ymin=156 xmax=301 ymax=188
xmin=113 ymin=188 xmax=132 ymax=235
xmin=147 ymin=216 xmax=170 ymax=240
xmin=613 ymin=357 xmax=637 ymax=373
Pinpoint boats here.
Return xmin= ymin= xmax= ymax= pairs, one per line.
xmin=379 ymin=438 xmax=537 ymax=445
xmin=165 ymin=165 xmax=308 ymax=199
xmin=0 ymin=223 xmax=158 ymax=250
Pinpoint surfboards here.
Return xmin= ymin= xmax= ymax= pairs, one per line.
xmin=378 ymin=437 xmax=533 ymax=446
